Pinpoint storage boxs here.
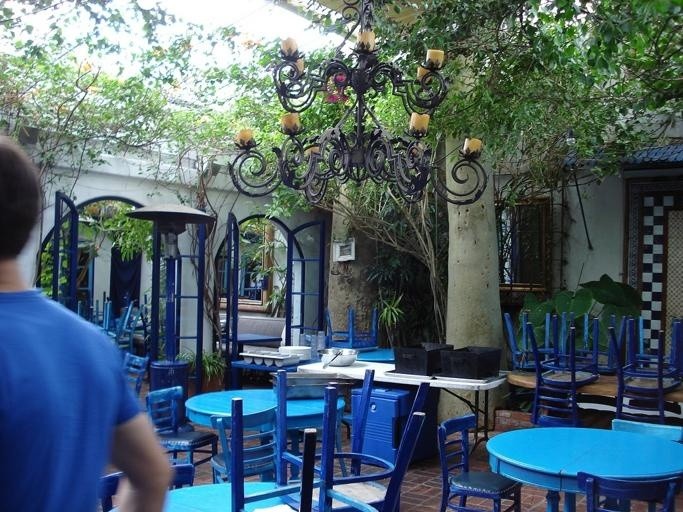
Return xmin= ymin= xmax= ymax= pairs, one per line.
xmin=392 ymin=342 xmax=456 ymax=376
xmin=440 ymin=346 xmax=505 ymax=380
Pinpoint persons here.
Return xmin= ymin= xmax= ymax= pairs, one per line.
xmin=1 ymin=137 xmax=173 ymax=512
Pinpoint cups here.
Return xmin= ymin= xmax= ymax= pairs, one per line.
xmin=318 ymin=332 xmax=324 ymax=348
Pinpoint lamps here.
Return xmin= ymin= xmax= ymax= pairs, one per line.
xmin=225 ymin=1 xmax=488 ymax=208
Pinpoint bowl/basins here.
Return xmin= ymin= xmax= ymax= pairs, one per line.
xmin=279 ymin=345 xmax=312 ymax=362
xmin=318 ymin=347 xmax=359 ymax=368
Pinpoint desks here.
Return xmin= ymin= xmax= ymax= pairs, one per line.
xmin=485 ymin=427 xmax=682 ymax=510
xmin=297 ymin=359 xmax=507 ymax=465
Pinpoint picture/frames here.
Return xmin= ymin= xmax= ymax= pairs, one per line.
xmin=332 ymin=239 xmax=356 ymax=263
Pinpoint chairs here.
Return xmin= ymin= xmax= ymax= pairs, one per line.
xmin=501 ymin=311 xmax=682 ymax=426
xmin=78 ymin=290 xmax=432 ymax=512
xmin=434 ymin=412 xmax=522 ymax=511
xmin=602 ymin=418 xmax=680 ymax=511
xmin=576 ymin=468 xmax=681 ymax=511
